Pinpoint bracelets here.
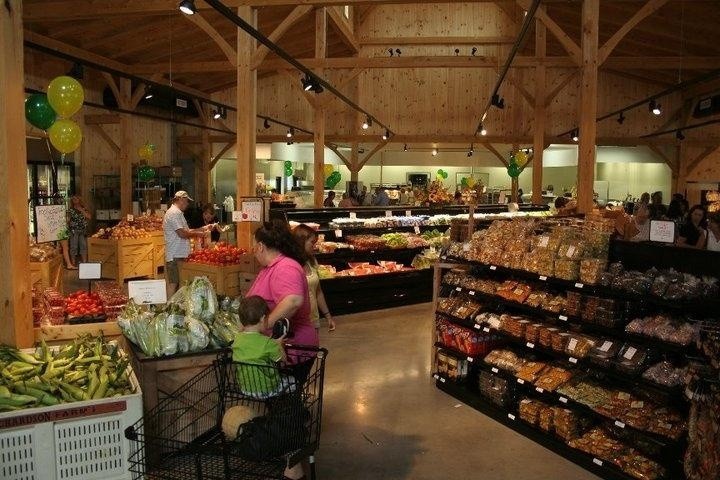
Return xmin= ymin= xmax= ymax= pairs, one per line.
xmin=322 ymin=311 xmax=331 ymax=315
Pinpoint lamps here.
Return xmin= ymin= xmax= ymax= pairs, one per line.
xmin=340 ymin=144 xmax=490 ymax=157
xmin=556 ymin=69 xmax=720 ymax=144
xmin=466 ymin=1 xmax=539 ymax=160
xmin=179 ymin=0 xmax=396 ymax=139
xmin=23 ymin=41 xmax=315 ymax=138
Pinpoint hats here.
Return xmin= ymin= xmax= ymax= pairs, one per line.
xmin=173 ymin=190 xmax=193 ymax=201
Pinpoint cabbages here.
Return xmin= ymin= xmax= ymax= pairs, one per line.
xmin=118 ymin=276 xmax=218 ymax=356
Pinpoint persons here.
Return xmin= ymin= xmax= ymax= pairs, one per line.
xmin=67 ymin=196 xmax=91 ymax=265
xmin=55 ymin=193 xmax=78 ymax=270
xmin=295 ymin=224 xmax=337 ymax=335
xmin=162 ymin=191 xmax=214 ymax=302
xmin=231 ymin=297 xmax=298 ymax=399
xmin=197 ymin=204 xmax=230 ymax=241
xmin=626 ymin=191 xmax=720 ymax=252
xmin=455 ymin=192 xmax=465 ymax=205
xmin=245 ymin=219 xmax=319 ymax=480
xmin=324 ymin=187 xmax=413 ymax=207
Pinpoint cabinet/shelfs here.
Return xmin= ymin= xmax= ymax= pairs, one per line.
xmin=269 ymin=206 xmax=549 ymax=320
xmin=429 ymin=241 xmax=719 ymax=480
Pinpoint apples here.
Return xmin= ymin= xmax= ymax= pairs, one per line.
xmin=91 ymin=224 xmax=152 ymax=241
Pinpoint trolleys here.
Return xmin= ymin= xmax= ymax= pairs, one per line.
xmin=122 ymin=337 xmax=331 ymax=479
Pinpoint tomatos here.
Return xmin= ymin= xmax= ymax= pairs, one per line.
xmin=185 ymin=241 xmax=254 ymax=264
xmin=63 ymin=291 xmax=102 ymax=322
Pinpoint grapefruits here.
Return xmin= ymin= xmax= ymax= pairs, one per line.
xmin=221 ymin=406 xmax=258 ymax=442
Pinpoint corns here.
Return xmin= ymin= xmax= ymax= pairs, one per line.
xmin=0 ymin=333 xmax=137 ymax=412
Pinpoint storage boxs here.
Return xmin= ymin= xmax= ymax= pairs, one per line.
xmin=1 ymin=337 xmax=146 ymax=480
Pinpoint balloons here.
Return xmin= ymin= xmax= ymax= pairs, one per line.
xmin=46 ymin=76 xmax=86 ymax=120
xmin=136 ymin=145 xmax=154 ymax=161
xmin=25 ymin=91 xmax=58 ymax=133
xmin=140 ymin=166 xmax=155 ymax=181
xmin=284 ymin=161 xmax=292 ymax=169
xmin=507 ymin=151 xmax=528 ymax=177
xmin=285 ymin=169 xmax=292 ymax=176
xmin=49 ymin=116 xmax=83 ymax=154
xmin=436 ymin=169 xmax=448 ymax=180
xmin=323 ymin=163 xmax=342 ymax=190
